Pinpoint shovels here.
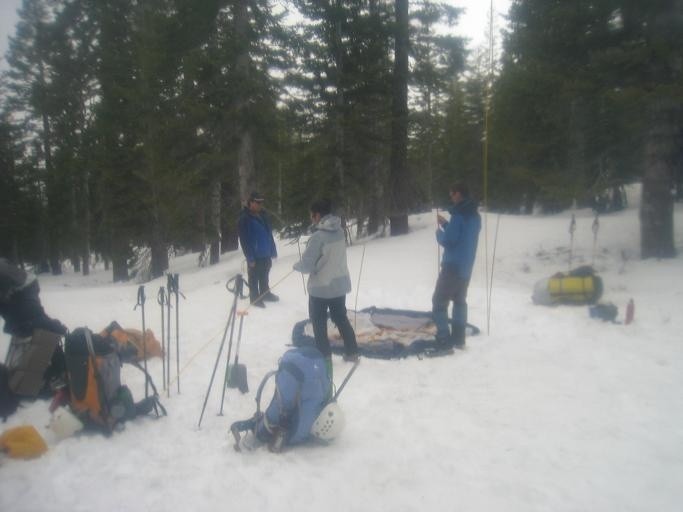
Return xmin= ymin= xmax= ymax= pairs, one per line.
xmin=226 ymin=311 xmax=248 ymax=395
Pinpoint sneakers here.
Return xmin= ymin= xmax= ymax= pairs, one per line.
xmin=425 ymin=347 xmax=454 ymax=357
xmin=342 ymin=355 xmax=358 ymax=361
xmin=250 ymin=298 xmax=265 ymax=308
xmin=261 ymin=292 xmax=279 ymax=302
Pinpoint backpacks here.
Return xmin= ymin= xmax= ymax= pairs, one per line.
xmin=64 ymin=326 xmax=138 ymax=439
xmin=0 ymin=361 xmax=25 ymax=424
xmin=232 ymin=345 xmax=331 ymax=452
xmin=547 ymin=266 xmax=603 ymax=304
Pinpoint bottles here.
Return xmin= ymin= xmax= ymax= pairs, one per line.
xmin=624 ymin=299 xmax=635 ymax=324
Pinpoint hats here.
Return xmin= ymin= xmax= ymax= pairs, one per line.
xmin=250 ymin=191 xmax=265 ymax=202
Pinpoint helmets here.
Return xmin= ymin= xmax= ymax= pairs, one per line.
xmin=312 ymin=402 xmax=345 ymax=441
xmin=48 ymin=405 xmax=84 ymax=438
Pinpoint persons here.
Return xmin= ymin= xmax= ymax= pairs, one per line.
xmin=240 ymin=189 xmax=279 ymax=308
xmin=293 ymin=198 xmax=359 ymax=362
xmin=426 ymin=182 xmax=483 ymax=357
xmin=0 ymin=254 xmax=66 ymax=373
